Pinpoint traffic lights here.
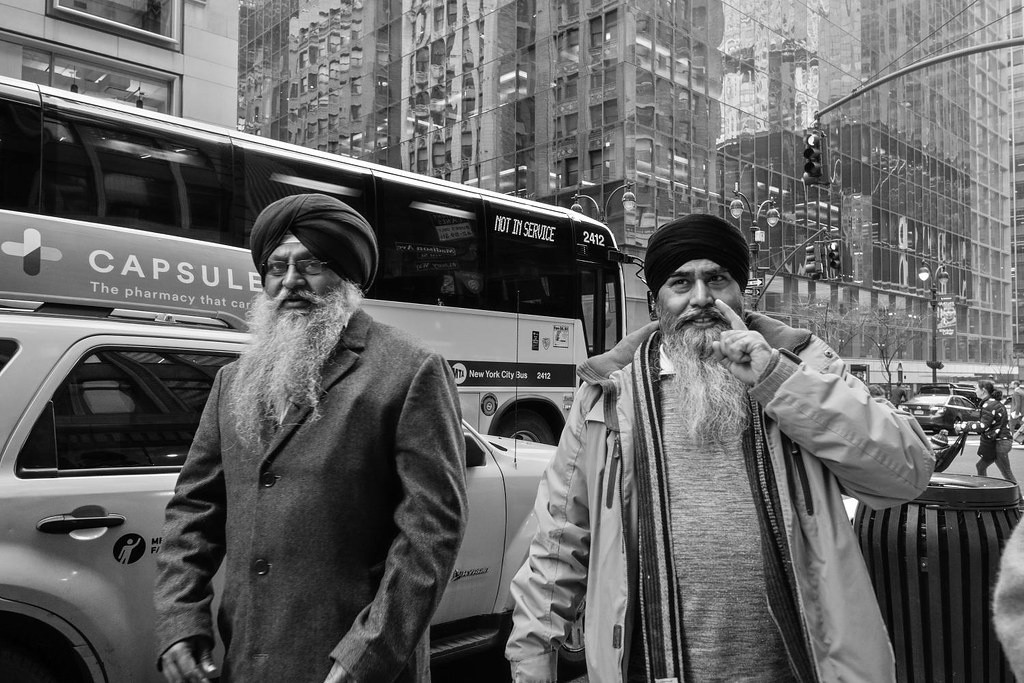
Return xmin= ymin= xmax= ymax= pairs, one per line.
xmin=805 ymin=242 xmax=823 ymax=279
xmin=826 ymin=240 xmax=842 ymax=280
xmin=803 ymin=130 xmax=829 ymax=188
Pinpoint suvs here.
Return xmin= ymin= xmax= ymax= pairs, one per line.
xmin=0 ymin=292 xmax=560 ymax=683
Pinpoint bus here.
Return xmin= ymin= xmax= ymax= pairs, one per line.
xmin=0 ymin=79 xmax=660 ymax=468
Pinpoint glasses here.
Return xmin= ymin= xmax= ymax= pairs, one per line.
xmin=260 ymin=259 xmax=327 ymax=276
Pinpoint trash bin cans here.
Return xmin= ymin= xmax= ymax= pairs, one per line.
xmin=854 ymin=473 xmax=1024 ymax=683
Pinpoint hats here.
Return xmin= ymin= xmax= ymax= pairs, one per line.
xmin=250 ymin=194 xmax=379 ymax=294
xmin=645 ymin=214 xmax=750 ymax=298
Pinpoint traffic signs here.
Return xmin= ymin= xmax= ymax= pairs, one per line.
xmin=745 ymin=278 xmax=764 ymax=299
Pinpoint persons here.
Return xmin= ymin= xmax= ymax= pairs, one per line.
xmin=955 ymin=380 xmax=1022 ymax=501
xmin=152 ymin=193 xmax=470 ymax=683
xmin=441 ymin=239 xmax=484 ymax=308
xmin=505 ymin=213 xmax=936 ymax=683
xmin=1010 ymin=381 xmax=1024 ymax=436
xmin=890 ymin=383 xmax=908 ymax=409
xmin=930 ymin=429 xmax=949 ymax=461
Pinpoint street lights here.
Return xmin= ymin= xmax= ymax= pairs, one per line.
xmin=570 ymin=179 xmax=638 ymax=229
xmin=731 ymin=191 xmax=779 ymax=309
xmin=918 ymin=259 xmax=950 ymax=383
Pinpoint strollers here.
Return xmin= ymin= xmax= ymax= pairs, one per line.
xmin=930 ymin=425 xmax=971 ymax=472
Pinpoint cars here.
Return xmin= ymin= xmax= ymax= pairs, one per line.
xmin=899 ymin=382 xmax=1012 ymax=436
